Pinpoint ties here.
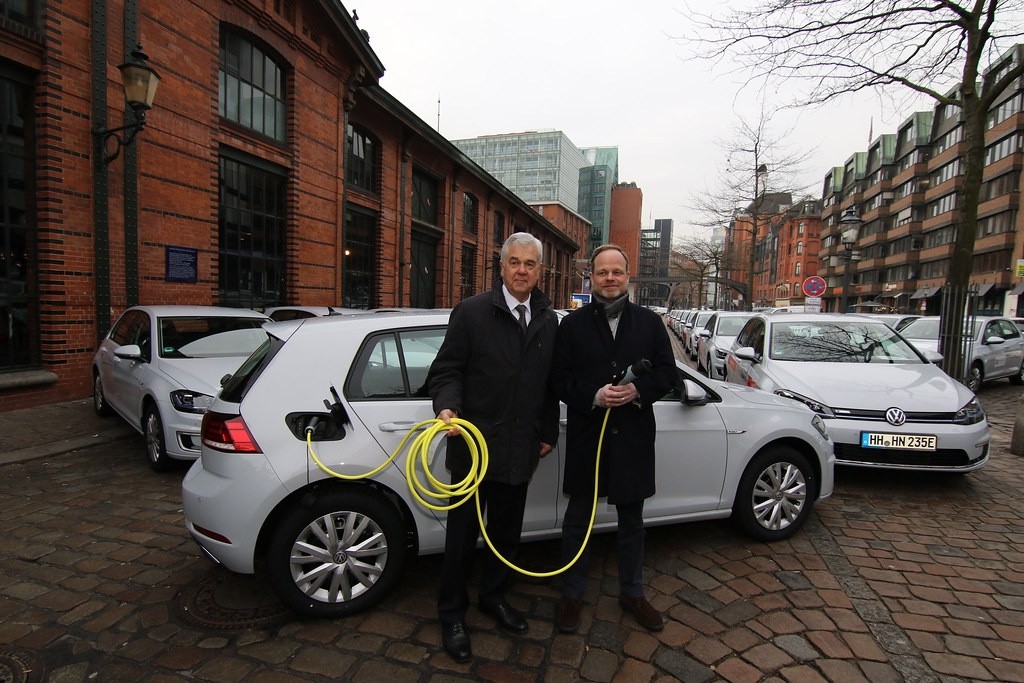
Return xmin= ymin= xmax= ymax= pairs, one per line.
xmin=515 ymin=305 xmax=528 ymax=335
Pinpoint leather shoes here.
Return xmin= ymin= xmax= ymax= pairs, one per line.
xmin=620 ymin=593 xmax=665 ymax=630
xmin=556 ymin=596 xmax=583 ymax=635
xmin=441 ymin=596 xmax=526 ymax=663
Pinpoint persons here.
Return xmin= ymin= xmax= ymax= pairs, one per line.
xmin=426 ymin=231 xmax=563 ymax=665
xmin=552 ymin=244 xmax=668 ymax=636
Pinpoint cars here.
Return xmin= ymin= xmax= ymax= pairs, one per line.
xmin=661 ymin=287 xmax=928 ymax=386
xmin=182 ymin=289 xmax=838 ymax=624
xmin=91 ymin=305 xmax=283 ymax=473
xmin=725 ymin=313 xmax=993 ymax=479
xmin=899 ymin=315 xmax=1024 ymax=395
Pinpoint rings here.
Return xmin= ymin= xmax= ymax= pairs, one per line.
xmin=623 ymin=397 xmax=625 ymax=402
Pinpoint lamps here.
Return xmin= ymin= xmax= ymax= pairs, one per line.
xmin=90 ymin=42 xmax=163 ymax=177
